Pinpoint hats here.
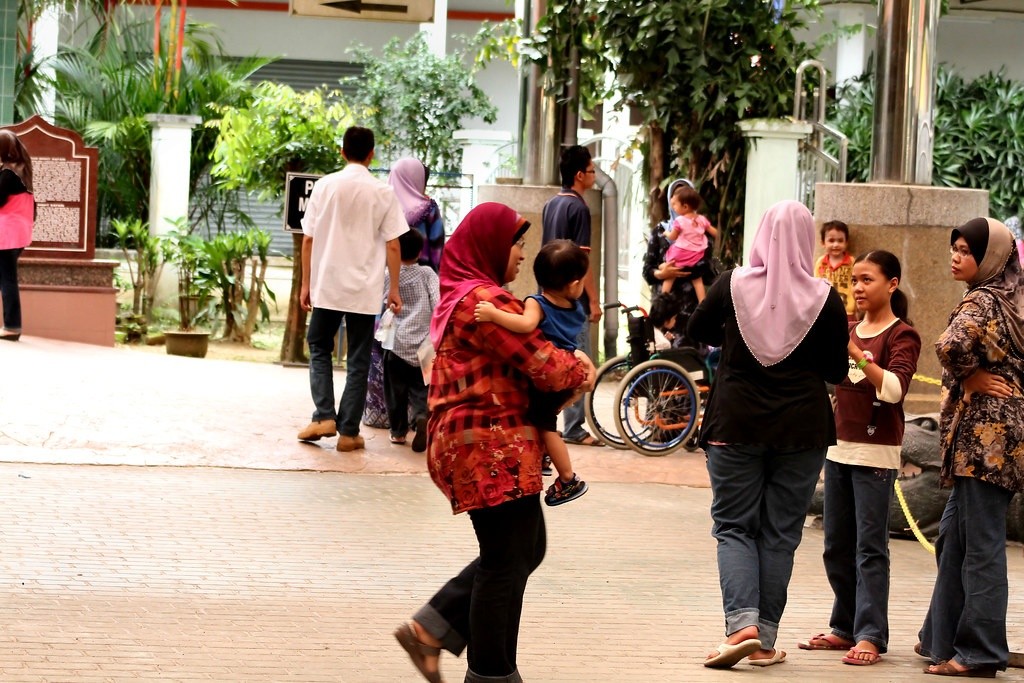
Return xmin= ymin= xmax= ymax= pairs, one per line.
xmin=510 ymin=212 xmax=532 ymax=249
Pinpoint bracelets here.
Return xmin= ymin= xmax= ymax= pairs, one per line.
xmin=857 ymin=349 xmax=874 ymax=369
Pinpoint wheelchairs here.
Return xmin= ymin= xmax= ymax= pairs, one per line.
xmin=584 ymin=301 xmax=714 ymax=457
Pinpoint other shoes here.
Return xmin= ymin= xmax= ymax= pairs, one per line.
xmin=297 ymin=420 xmax=337 ymax=441
xmin=337 ymin=435 xmax=365 ymax=452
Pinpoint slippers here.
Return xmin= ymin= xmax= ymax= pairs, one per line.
xmin=0 ymin=329 xmax=22 ymax=340
xmin=702 ymin=638 xmax=762 ymax=668
xmin=797 ymin=634 xmax=857 ymax=650
xmin=389 ymin=431 xmax=407 ymax=444
xmin=749 ymin=647 xmax=787 ymax=666
xmin=411 ymin=416 xmax=427 ymax=453
xmin=562 ymin=431 xmax=607 ymax=446
xmin=843 ymin=647 xmax=882 ymax=665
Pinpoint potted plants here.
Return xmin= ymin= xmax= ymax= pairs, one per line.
xmin=167 ymin=230 xmax=211 ymax=357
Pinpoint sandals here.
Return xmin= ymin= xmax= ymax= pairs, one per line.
xmin=538 ymin=455 xmax=553 ymax=475
xmin=544 ymin=473 xmax=589 ymax=506
xmin=923 ymin=656 xmax=998 ymax=678
xmin=392 ymin=621 xmax=444 ymax=683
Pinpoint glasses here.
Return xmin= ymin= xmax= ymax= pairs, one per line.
xmin=948 ymin=244 xmax=972 ymax=260
xmin=582 ymin=170 xmax=596 ymax=174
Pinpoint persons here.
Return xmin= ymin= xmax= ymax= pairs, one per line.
xmin=362 ymin=157 xmax=445 ymax=427
xmin=914 ymin=218 xmax=1024 ymax=678
xmin=298 ymin=127 xmax=410 ymax=452
xmin=474 ymin=239 xmax=590 ymax=506
xmin=643 ymin=178 xmax=718 ymax=416
xmin=813 ymin=220 xmax=863 ymax=321
xmin=798 ymin=249 xmax=921 ymax=666
xmin=686 ymin=200 xmax=850 ymax=668
xmin=537 ymin=146 xmax=606 ymax=447
xmin=394 ymin=203 xmax=597 ymax=683
xmin=374 ymin=227 xmax=442 ymax=453
xmin=0 ymin=128 xmax=34 ymax=341
